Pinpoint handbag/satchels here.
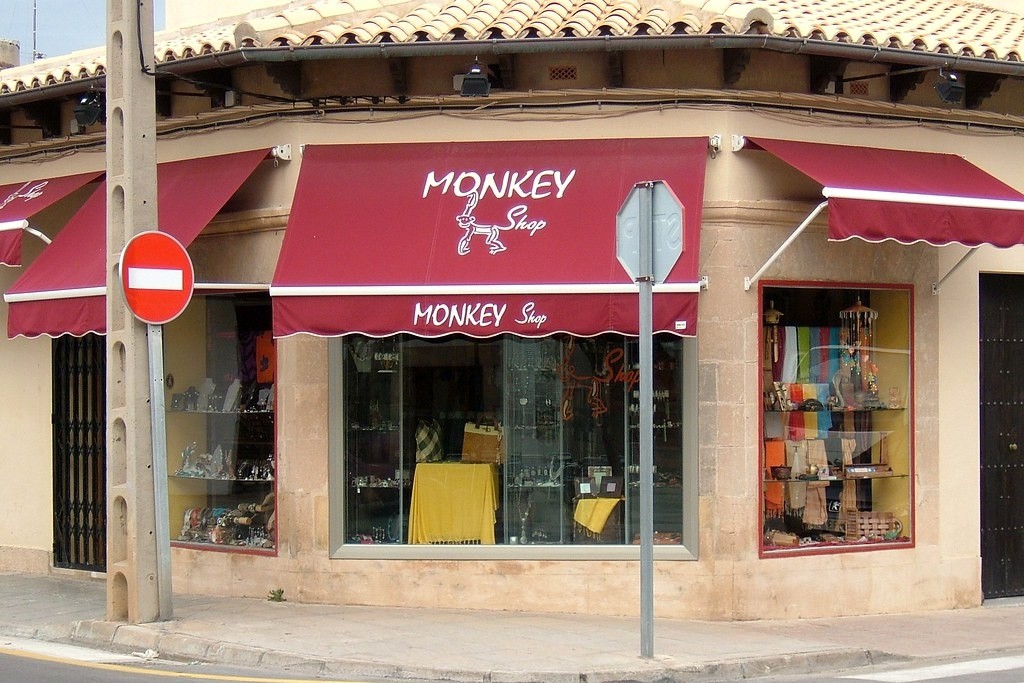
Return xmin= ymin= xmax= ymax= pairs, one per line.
xmin=462 ymin=413 xmax=505 ymax=464
xmin=416 ymin=416 xmax=444 ymax=462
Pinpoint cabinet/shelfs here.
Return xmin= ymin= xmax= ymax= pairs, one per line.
xmin=344 ymin=333 xmax=412 ymax=546
xmin=762 ymin=407 xmax=908 ymax=482
xmin=503 ymin=334 xmax=585 ymax=544
xmin=623 ymin=335 xmax=684 ymax=545
xmin=165 ymin=409 xmax=276 ymax=482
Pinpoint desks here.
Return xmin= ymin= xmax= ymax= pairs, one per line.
xmin=415 ymin=462 xmax=498 ymax=546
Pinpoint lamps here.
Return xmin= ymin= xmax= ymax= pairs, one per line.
xmin=839 ymin=60 xmax=965 ymax=104
xmin=73 ymin=87 xmax=171 ymax=126
xmin=460 ymin=54 xmax=498 ymax=97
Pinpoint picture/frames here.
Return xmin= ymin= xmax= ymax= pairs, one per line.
xmin=588 ymin=466 xmax=613 ymax=492
